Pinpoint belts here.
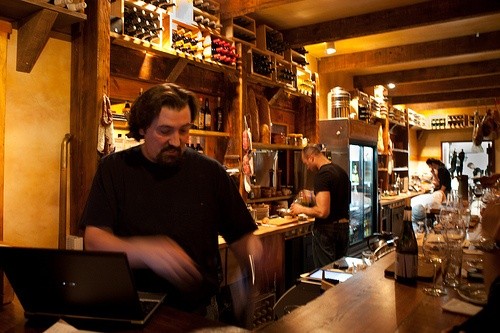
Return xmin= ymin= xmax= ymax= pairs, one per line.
xmin=333 ymin=218 xmax=349 ymax=223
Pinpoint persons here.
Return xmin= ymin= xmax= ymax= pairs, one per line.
xmin=290 ymin=145 xmax=352 ymax=269
xmin=424 ymin=157 xmax=482 ymax=197
xmin=77 ymin=83 xmax=270 ymax=325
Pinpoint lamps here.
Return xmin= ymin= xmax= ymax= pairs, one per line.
xmin=326 ymin=42 xmax=336 ymax=54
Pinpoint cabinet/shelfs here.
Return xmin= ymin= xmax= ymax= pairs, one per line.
xmin=316 ymin=117 xmax=378 ymax=257
xmin=350 ymin=89 xmax=369 ymax=119
xmin=378 ymin=185 xmax=440 ymax=242
xmin=408 ymin=108 xmax=427 ymax=130
xmin=371 ymin=115 xmax=409 ymax=196
xmin=71 ymin=0 xmax=321 ymax=205
xmin=389 ymin=107 xmax=406 ymax=126
xmin=369 ymin=96 xmax=389 ymax=119
xmin=71 ymin=212 xmax=315 ymax=333
xmin=467 ymin=114 xmax=485 ymax=128
xmin=446 ymin=114 xmax=466 ymax=129
xmin=246 ymin=293 xmax=277 ymax=332
xmin=427 ymin=115 xmax=446 ymax=130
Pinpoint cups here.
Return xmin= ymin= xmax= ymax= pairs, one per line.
xmin=446 ymin=239 xmax=463 ymax=287
xmin=439 ymin=208 xmax=470 ymax=232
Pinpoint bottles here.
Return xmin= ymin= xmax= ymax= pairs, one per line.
xmin=115 ymin=134 xmax=145 ymax=152
xmin=138 ymin=9 xmax=165 ymax=42
xmin=297 ymin=79 xmax=315 ymax=90
xmin=277 ymin=68 xmax=297 ymax=84
xmin=293 ymin=45 xmax=310 ymax=66
xmin=172 ymin=28 xmax=212 ymax=60
xmin=395 ymin=206 xmax=418 ymax=288
xmin=266 ymin=31 xmax=288 ymax=54
xmin=233 ymin=30 xmax=256 ymax=43
xmin=141 ymin=0 xmax=218 ymax=28
xmin=124 ymin=7 xmax=145 ymax=39
xmin=432 ymin=115 xmax=463 ymax=129
xmin=253 ymin=54 xmax=276 ymax=75
xmin=138 ymin=88 xmax=143 ymax=96
xmin=390 ymin=181 xmax=400 ymax=195
xmin=359 ymin=97 xmax=370 ymax=120
xmin=234 ymin=16 xmax=252 ymax=27
xmin=185 ymin=134 xmax=204 ymax=154
xmin=211 ymin=38 xmax=239 ymax=65
xmin=192 ymin=97 xmax=224 ymax=132
xmin=47 ymin=0 xmax=87 ymax=12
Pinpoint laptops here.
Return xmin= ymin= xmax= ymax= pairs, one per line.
xmin=1 ymin=244 xmax=171 ymax=330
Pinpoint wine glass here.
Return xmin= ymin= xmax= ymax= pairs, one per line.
xmin=436 ymin=214 xmax=466 ymax=294
xmin=422 ymin=228 xmax=448 ymax=296
xmin=473 ymin=187 xmax=482 ymax=208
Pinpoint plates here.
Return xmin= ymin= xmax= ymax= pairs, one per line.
xmin=458 ymin=285 xmax=489 ymax=304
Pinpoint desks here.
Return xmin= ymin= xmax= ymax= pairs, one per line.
xmin=299 ymin=256 xmax=367 ymax=288
